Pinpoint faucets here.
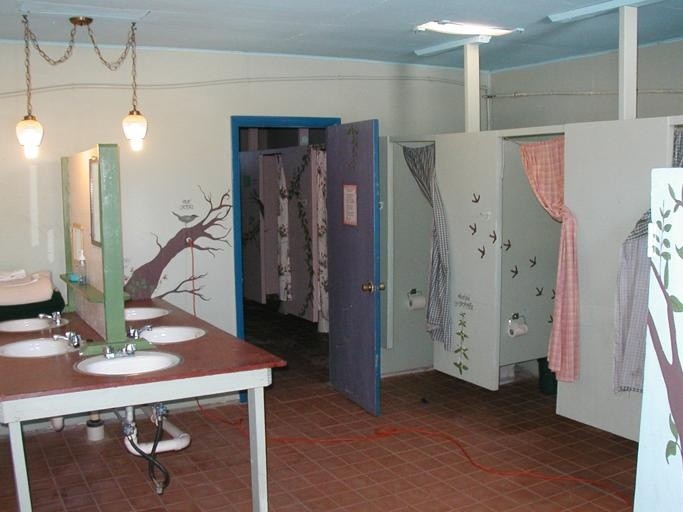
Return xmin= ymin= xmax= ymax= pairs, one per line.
xmin=38 ymin=309 xmax=64 ymax=325
xmin=121 ymin=343 xmax=137 ymax=355
xmin=53 ymin=330 xmax=84 ymax=347
xmin=124 ymin=325 xmax=154 ymax=338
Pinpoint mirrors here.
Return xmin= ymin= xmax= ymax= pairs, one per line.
xmin=68 ymin=147 xmax=103 ymax=295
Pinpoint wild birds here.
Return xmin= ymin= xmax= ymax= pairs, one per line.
xmin=172 ymin=211 xmax=199 ymax=227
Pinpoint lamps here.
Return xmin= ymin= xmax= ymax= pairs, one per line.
xmin=16 ymin=17 xmax=147 ymax=158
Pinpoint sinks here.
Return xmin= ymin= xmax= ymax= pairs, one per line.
xmin=75 ymin=350 xmax=181 ymax=375
xmin=0 ymin=338 xmax=74 ymax=358
xmin=125 ymin=304 xmax=171 ymax=321
xmin=142 ymin=325 xmax=207 ymax=346
xmin=0 ymin=316 xmax=71 ymax=332
